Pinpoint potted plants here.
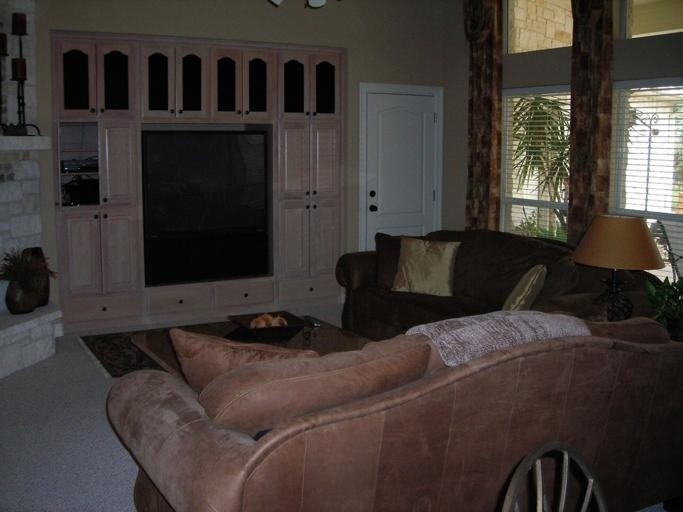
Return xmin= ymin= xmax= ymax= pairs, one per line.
xmin=0 ymin=246 xmax=61 ymax=315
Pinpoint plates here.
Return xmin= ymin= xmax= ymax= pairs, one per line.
xmin=227 ymin=310 xmax=305 ymax=341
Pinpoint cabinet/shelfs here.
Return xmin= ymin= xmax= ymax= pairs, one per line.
xmin=272 ymin=120 xmax=345 ymax=309
xmin=138 ymin=33 xmax=208 ymax=123
xmin=273 ymin=43 xmax=347 ymax=120
xmin=50 ymin=29 xmax=138 ymax=120
xmin=207 ymin=38 xmax=273 ymax=123
xmin=53 ymin=119 xmax=145 ymax=336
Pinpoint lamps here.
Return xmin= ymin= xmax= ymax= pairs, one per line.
xmin=267 ymin=0 xmax=342 ymax=9
xmin=572 ymin=213 xmax=666 ymax=322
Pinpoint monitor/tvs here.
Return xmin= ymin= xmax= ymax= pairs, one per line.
xmin=140 ymin=122 xmax=274 ymax=234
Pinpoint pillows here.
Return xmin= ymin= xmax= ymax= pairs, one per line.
xmin=502 ymin=264 xmax=547 ymax=311
xmin=390 ymin=236 xmax=462 ymax=297
xmin=375 ymin=232 xmax=439 ymax=297
xmin=531 ymin=256 xmax=578 ymax=312
xmin=169 ymin=326 xmax=319 ymax=391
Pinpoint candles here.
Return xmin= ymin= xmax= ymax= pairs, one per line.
xmin=12 ymin=58 xmax=26 ymax=80
xmin=12 ymin=13 xmax=26 ymax=34
xmin=0 ymin=33 xmax=7 ymax=54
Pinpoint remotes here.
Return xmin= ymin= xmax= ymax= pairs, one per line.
xmin=306 ymin=314 xmax=321 ymax=328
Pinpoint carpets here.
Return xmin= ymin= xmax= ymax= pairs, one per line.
xmin=77 ymin=315 xmax=377 ymax=379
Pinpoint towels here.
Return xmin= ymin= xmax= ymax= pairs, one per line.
xmin=405 ymin=309 xmax=591 ymax=368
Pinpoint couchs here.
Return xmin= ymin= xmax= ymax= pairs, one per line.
xmin=106 ymin=310 xmax=683 ymax=512
xmin=335 ymin=230 xmax=678 ymax=342
